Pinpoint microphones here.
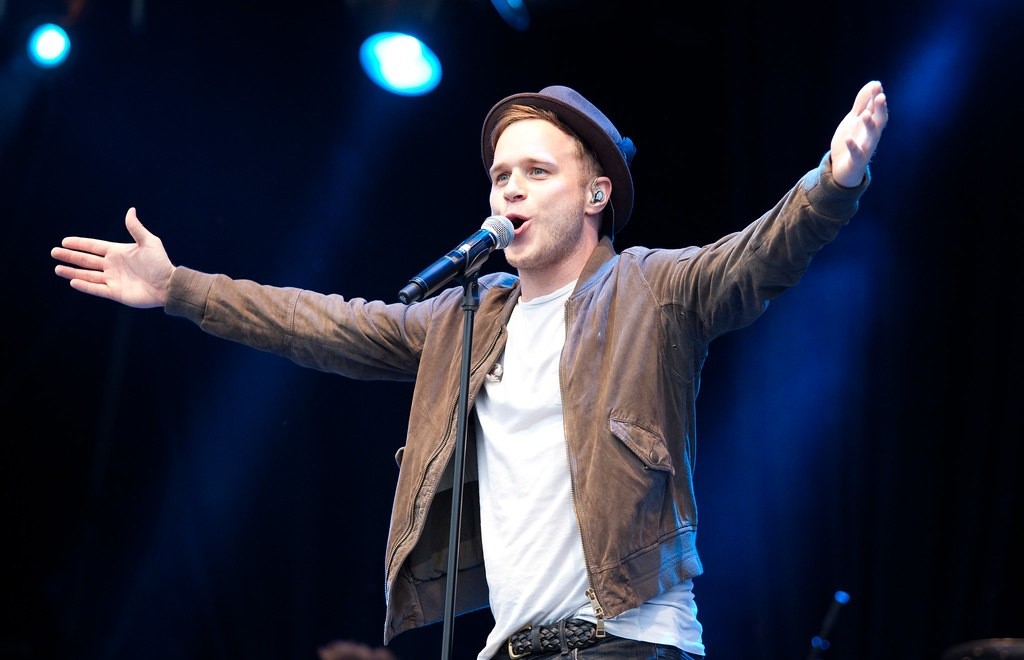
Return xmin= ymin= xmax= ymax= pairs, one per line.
xmin=399 ymin=215 xmax=515 ymax=305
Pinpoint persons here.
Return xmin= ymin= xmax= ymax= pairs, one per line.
xmin=52 ymin=79 xmax=888 ymax=660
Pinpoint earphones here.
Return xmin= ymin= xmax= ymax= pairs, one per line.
xmin=592 ymin=188 xmax=604 ymax=204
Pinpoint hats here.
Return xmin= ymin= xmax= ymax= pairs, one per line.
xmin=480 ymin=85 xmax=634 ymax=233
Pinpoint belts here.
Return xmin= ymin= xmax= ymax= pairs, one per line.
xmin=499 ymin=617 xmax=617 ymax=660
